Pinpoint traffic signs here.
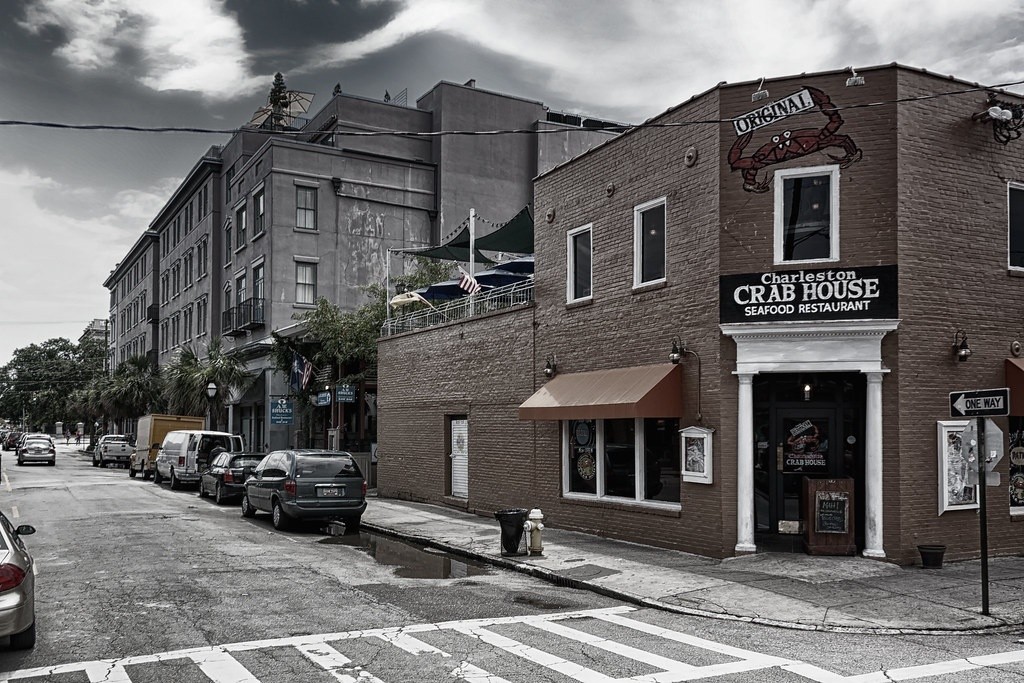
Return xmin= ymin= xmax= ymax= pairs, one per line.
xmin=948 ymin=387 xmax=1011 ymax=419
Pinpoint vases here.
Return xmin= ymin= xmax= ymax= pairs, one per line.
xmin=918 ymin=544 xmax=947 ymax=568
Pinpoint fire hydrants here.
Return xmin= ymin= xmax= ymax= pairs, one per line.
xmin=523 ymin=506 xmax=546 ymax=556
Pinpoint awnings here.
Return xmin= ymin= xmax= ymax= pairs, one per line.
xmin=221 ymin=368 xmax=264 ymax=406
xmin=1006 ymin=357 xmax=1024 ymax=416
xmin=517 ymin=364 xmax=684 ymax=418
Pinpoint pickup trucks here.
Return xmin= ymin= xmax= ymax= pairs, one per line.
xmin=92 ymin=434 xmax=137 ymax=469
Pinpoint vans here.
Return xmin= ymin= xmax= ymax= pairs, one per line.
xmin=151 ymin=428 xmax=245 ymax=490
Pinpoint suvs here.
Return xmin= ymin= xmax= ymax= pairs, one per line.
xmin=239 ymin=448 xmax=368 ymax=531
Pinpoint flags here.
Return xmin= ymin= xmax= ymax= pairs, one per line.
xmin=302 ymin=357 xmax=312 ymax=389
xmin=459 ymin=273 xmax=481 ymax=294
xmin=288 ymin=352 xmax=305 ymax=391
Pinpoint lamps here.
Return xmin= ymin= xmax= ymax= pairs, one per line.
xmin=839 ymin=65 xmax=866 ymax=88
xmin=750 ymin=77 xmax=770 ymax=104
xmin=668 ymin=334 xmax=685 ymax=364
xmin=951 ymin=330 xmax=973 ymax=361
xmin=543 ymin=359 xmax=558 ymax=377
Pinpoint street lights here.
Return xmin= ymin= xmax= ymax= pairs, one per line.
xmin=206 ymin=380 xmax=218 ymax=431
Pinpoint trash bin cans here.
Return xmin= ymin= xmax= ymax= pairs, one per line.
xmin=494 ymin=508 xmax=528 ymax=557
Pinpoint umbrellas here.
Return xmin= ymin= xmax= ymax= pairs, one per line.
xmin=389 ymin=254 xmax=534 ymax=331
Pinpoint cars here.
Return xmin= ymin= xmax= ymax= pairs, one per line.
xmin=0 ymin=426 xmax=57 ymax=467
xmin=197 ymin=450 xmax=270 ymax=506
xmin=569 ymin=443 xmax=785 ymax=534
xmin=0 ymin=509 xmax=37 ymax=651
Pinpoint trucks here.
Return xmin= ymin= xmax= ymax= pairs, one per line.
xmin=127 ymin=414 xmax=206 ymax=481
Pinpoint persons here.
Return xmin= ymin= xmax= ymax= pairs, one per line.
xmin=76 ymin=429 xmax=81 ymax=443
xmin=65 ymin=429 xmax=71 ymax=444
